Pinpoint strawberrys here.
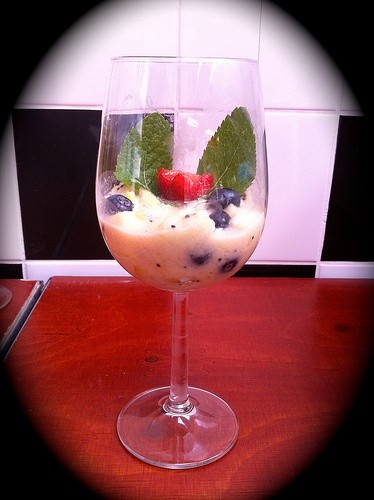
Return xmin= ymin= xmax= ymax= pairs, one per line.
xmin=157 ymin=168 xmax=213 ymax=206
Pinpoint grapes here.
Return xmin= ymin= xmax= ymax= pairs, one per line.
xmin=100 ymin=169 xmax=132 ymax=217
xmin=207 ymin=187 xmax=240 ymax=228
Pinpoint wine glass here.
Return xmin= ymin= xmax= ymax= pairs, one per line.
xmin=95 ymin=57 xmax=269 ymax=469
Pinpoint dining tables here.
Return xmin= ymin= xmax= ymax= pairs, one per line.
xmin=4 ymin=275 xmax=374 ymax=500
